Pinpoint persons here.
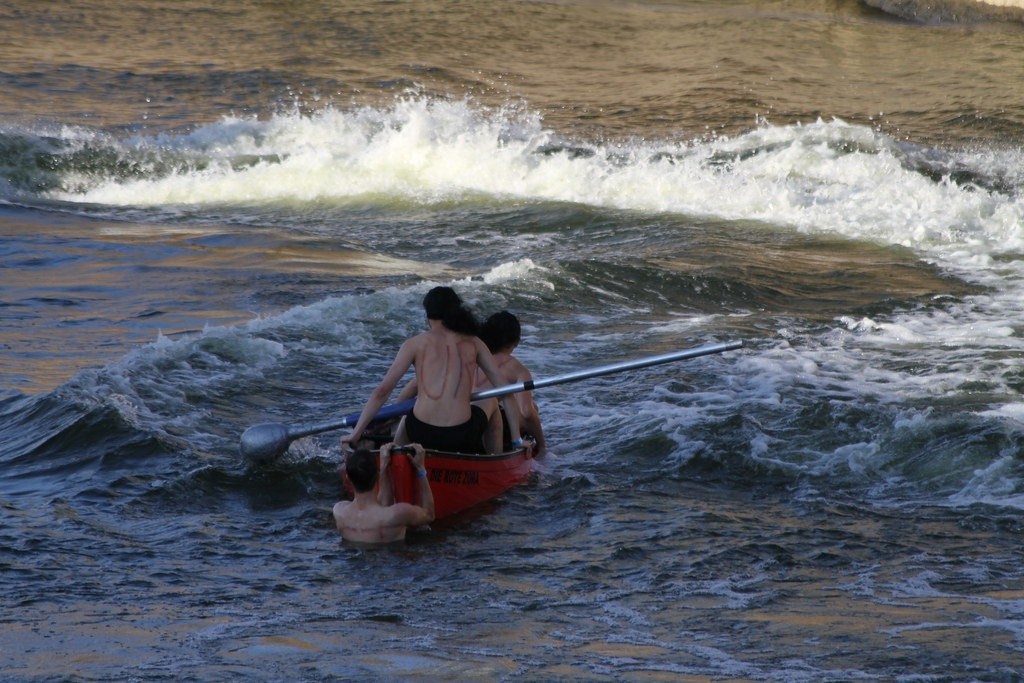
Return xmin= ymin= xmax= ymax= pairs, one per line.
xmin=331 ymin=444 xmax=435 ymax=543
xmin=341 ymin=286 xmax=545 ymax=459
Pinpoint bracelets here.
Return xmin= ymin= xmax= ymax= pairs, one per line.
xmin=513 ymin=437 xmax=523 ymax=446
xmin=419 ymin=470 xmax=427 ymax=478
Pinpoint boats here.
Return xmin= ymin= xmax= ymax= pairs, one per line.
xmin=340 ymin=417 xmax=538 ymax=531
